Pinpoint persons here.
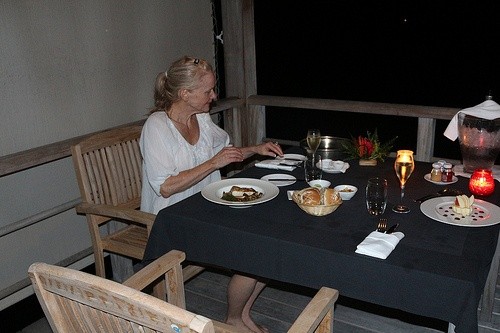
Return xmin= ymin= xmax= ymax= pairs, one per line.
xmin=139 ymin=55 xmax=284 ymax=333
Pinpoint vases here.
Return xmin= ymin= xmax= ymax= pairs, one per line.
xmin=358 ymin=158 xmax=377 ymax=166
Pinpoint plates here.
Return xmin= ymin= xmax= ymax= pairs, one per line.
xmin=419 ymin=196 xmax=500 ymax=227
xmin=200 ymin=178 xmax=280 ymax=208
xmin=261 ymin=173 xmax=296 ymax=187
xmin=315 ymin=159 xmax=350 ymax=173
xmin=276 ymin=154 xmax=308 ymax=162
xmin=424 ymin=173 xmax=459 ymax=185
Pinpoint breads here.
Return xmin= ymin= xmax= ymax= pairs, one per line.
xmin=293 ymin=187 xmax=342 ymax=206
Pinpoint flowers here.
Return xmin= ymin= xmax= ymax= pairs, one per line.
xmin=338 ymin=129 xmax=398 ymax=163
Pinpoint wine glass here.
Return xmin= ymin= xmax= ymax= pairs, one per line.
xmin=306 ymin=130 xmax=322 ymax=155
xmin=392 ymin=150 xmax=415 ymax=213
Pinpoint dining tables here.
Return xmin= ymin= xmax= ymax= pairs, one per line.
xmin=143 ymin=143 xmax=500 ymax=331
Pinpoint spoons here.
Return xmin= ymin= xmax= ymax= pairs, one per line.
xmin=279 ymin=163 xmax=303 ymax=169
xmin=283 ymin=158 xmax=305 ymax=163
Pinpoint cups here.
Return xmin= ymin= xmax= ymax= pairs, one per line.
xmin=457 ymin=111 xmax=500 ymax=174
xmin=304 ymin=154 xmax=322 ymax=183
xmin=468 ymin=168 xmax=495 ymax=196
xmin=366 ymin=178 xmax=388 ymax=216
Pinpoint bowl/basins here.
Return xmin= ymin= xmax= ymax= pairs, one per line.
xmin=334 ymin=184 xmax=358 ymax=200
xmin=309 ymin=180 xmax=331 ymax=189
xmin=299 ymin=136 xmax=356 ymax=162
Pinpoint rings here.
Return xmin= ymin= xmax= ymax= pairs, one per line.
xmin=274 ymin=141 xmax=279 ymax=146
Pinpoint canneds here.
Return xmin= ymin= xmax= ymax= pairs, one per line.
xmin=431 ymin=160 xmax=452 ymax=182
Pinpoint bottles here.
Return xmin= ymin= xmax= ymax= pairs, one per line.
xmin=430 ymin=163 xmax=442 ymax=182
xmin=441 ymin=163 xmax=452 ymax=182
xmin=438 ymin=160 xmax=447 ymax=172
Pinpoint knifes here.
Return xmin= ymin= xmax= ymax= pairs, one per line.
xmin=385 ymin=223 xmax=400 ymax=235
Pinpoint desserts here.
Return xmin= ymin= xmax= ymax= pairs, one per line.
xmin=452 ymin=194 xmax=475 ymax=218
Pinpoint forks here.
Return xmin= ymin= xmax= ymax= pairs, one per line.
xmin=377 ymin=218 xmax=387 ymax=233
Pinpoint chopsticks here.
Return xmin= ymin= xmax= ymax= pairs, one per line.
xmin=268 ymin=178 xmax=307 ymax=182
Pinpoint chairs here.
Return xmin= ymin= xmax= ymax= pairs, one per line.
xmin=26 ymin=246 xmax=340 ymax=333
xmin=70 ymin=124 xmax=207 ymax=298
